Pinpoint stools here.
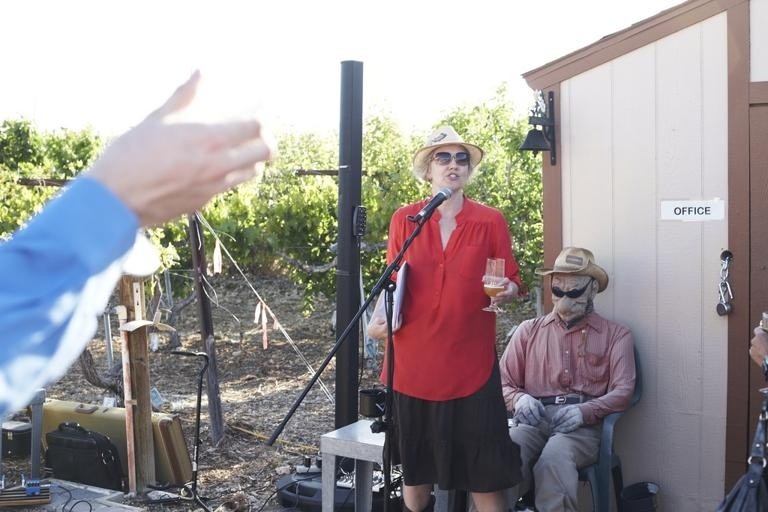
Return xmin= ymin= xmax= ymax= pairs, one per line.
xmin=321 ymin=417 xmax=458 ymax=511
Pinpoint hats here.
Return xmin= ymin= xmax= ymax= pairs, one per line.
xmin=412 ymin=125 xmax=483 ymax=182
xmin=535 ymin=246 xmax=608 ymax=293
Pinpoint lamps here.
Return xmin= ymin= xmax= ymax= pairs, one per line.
xmin=519 ymin=92 xmax=556 ymax=166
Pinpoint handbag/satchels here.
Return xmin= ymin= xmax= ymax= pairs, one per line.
xmin=46 ymin=422 xmax=122 ymax=491
xmin=714 ymin=464 xmax=768 ymax=512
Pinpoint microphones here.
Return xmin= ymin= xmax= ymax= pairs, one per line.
xmin=413 ymin=187 xmax=452 ymax=222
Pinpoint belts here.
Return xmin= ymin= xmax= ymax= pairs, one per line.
xmin=530 ymin=395 xmax=590 ymax=405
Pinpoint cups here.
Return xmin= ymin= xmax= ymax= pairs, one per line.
xmin=358 ymin=388 xmax=384 ymax=417
xmin=621 ymin=480 xmax=662 ymax=512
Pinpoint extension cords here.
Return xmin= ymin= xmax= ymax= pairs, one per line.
xmin=296 ymin=465 xmax=322 ymax=473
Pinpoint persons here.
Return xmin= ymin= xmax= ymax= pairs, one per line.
xmin=0 ymin=67 xmax=274 ymax=428
xmin=367 ymin=122 xmax=525 ymax=511
xmin=497 ymin=245 xmax=639 ymax=512
xmin=716 ymin=310 xmax=768 ymax=512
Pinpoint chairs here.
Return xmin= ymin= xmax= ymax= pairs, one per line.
xmin=576 ymin=343 xmax=642 ymax=512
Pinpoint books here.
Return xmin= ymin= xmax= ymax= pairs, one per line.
xmin=366 ymin=261 xmax=410 ymax=345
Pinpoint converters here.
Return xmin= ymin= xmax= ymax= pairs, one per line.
xmin=316 ymin=457 xmax=322 ymax=468
xmin=304 ymin=455 xmax=311 ymax=467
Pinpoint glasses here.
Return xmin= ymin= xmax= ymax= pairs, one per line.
xmin=430 ymin=153 xmax=469 ymax=165
xmin=547 ymin=273 xmax=593 ymax=299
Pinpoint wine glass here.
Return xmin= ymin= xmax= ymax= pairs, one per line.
xmin=481 ymin=259 xmax=506 ymax=314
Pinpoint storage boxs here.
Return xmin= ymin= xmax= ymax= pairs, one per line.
xmin=1 ymin=419 xmax=32 ymax=458
